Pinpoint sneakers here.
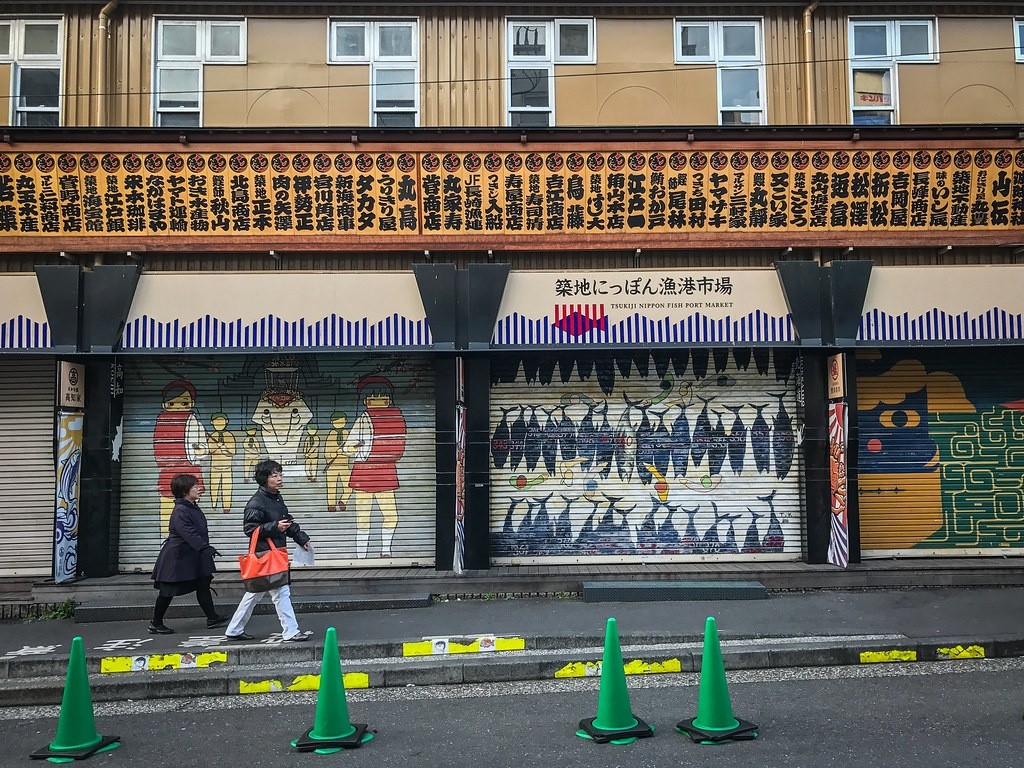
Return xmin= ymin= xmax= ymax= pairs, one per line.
xmin=148 ymin=621 xmax=174 ymax=634
xmin=207 ymin=615 xmax=231 ymax=629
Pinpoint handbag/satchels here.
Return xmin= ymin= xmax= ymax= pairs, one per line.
xmin=240 ymin=526 xmax=290 ymax=593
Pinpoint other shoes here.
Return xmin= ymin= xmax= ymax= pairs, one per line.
xmin=228 ymin=632 xmax=254 ymax=641
xmin=290 ymin=632 xmax=309 ymax=641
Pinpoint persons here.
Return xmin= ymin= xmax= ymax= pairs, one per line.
xmin=225 ymin=460 xmax=310 ymax=641
xmin=148 ymin=473 xmax=230 ymax=634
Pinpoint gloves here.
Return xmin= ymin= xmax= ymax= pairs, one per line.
xmin=203 ymin=546 xmax=220 ymax=558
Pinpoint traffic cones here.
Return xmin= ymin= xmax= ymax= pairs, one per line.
xmin=289 ymin=628 xmax=376 ymax=756
xmin=575 ymin=617 xmax=657 ymax=746
xmin=672 ymin=616 xmax=759 ymax=746
xmin=27 ymin=636 xmax=123 ymax=764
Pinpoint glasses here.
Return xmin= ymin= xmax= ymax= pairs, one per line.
xmin=268 ymin=473 xmax=284 ymax=478
